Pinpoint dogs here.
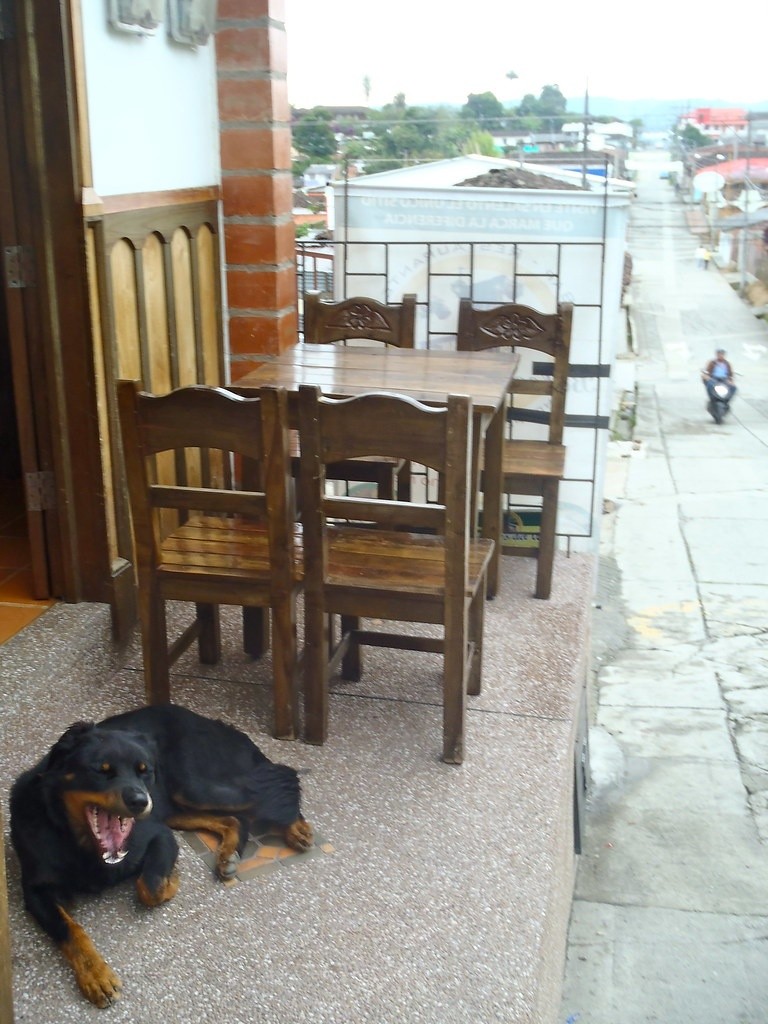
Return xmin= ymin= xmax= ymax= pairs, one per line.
xmin=8 ymin=700 xmax=314 ymax=1008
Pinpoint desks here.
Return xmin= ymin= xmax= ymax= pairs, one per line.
xmin=226 ymin=338 xmax=521 ymax=600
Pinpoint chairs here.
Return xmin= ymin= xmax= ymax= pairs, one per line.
xmin=113 ymin=378 xmax=298 ymax=743
xmin=438 ymin=297 xmax=573 ymax=598
xmin=298 ymin=383 xmax=497 ymax=765
xmin=287 ymin=291 xmax=418 ymax=500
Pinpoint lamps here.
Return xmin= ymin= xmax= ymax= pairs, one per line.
xmin=167 ymin=1 xmax=218 ymax=45
xmin=110 ymin=0 xmax=164 ymax=35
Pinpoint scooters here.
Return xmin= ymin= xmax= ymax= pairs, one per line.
xmin=702 ymin=370 xmax=738 ymax=425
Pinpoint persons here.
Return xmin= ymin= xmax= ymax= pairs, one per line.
xmin=703 ymin=348 xmax=736 ymax=408
xmin=694 ymin=245 xmax=711 ymax=270
